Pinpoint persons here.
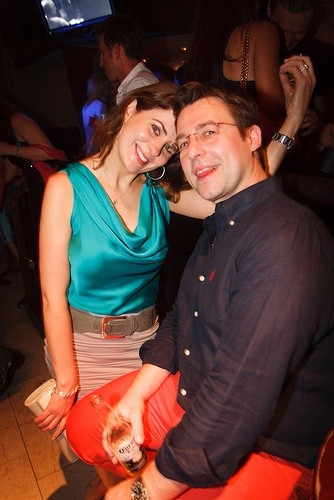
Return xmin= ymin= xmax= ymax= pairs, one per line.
xmin=0 ymin=94 xmax=57 ymax=270
xmin=39 ymin=0 xmax=334 ymax=500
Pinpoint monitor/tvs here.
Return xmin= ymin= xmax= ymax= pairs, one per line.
xmin=37 ymin=0 xmax=116 ymax=35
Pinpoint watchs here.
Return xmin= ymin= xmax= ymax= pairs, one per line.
xmin=129 ymin=472 xmax=149 ymax=500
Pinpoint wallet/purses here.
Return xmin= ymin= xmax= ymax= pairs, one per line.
xmin=23 ymin=378 xmax=79 ymax=464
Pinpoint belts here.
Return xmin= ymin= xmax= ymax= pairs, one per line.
xmin=67 ymin=305 xmax=158 ymax=340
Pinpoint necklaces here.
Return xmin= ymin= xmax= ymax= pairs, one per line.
xmin=101 ymin=153 xmax=135 ymax=206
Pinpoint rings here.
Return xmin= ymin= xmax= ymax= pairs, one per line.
xmin=299 ymin=64 xmax=309 ymax=72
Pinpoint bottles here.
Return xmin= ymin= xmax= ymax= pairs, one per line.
xmin=89 ymin=393 xmax=148 ymax=474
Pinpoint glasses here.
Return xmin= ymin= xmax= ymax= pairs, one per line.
xmin=174 ymin=121 xmax=238 ymax=154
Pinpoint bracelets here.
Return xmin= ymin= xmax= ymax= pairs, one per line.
xmin=272 ymin=131 xmax=295 ymax=150
xmin=16 ymin=143 xmax=23 ymax=158
xmin=53 ymin=384 xmax=80 ymax=398
